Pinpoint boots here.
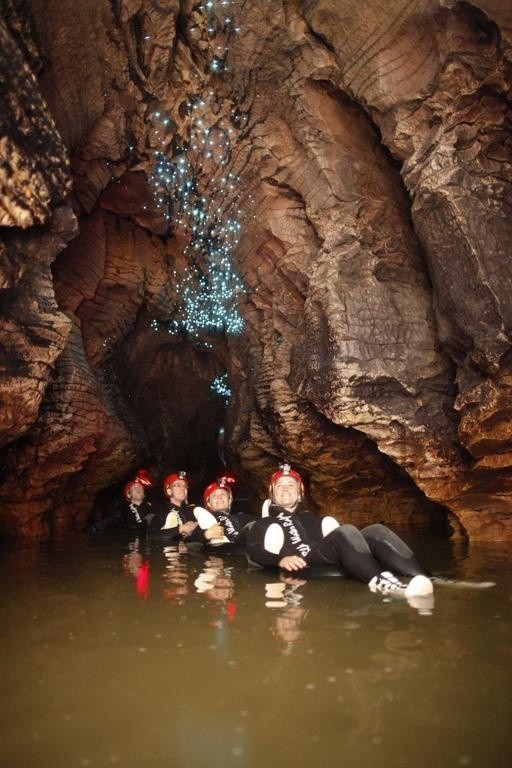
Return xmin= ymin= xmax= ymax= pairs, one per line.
xmin=368 ymin=570 xmax=434 ymax=598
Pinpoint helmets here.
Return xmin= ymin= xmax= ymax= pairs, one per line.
xmin=203 ymin=477 xmax=235 ymax=512
xmin=269 ymin=462 xmax=305 ymax=501
xmin=124 ymin=480 xmax=145 ymax=502
xmin=164 ymin=472 xmax=190 ymax=489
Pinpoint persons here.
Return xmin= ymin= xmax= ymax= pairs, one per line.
xmin=241 ymin=462 xmax=496 ymax=600
xmin=151 ymin=471 xmax=272 ymax=544
xmin=179 ymin=482 xmax=341 ymax=558
xmin=122 ymin=468 xmax=181 ymax=534
